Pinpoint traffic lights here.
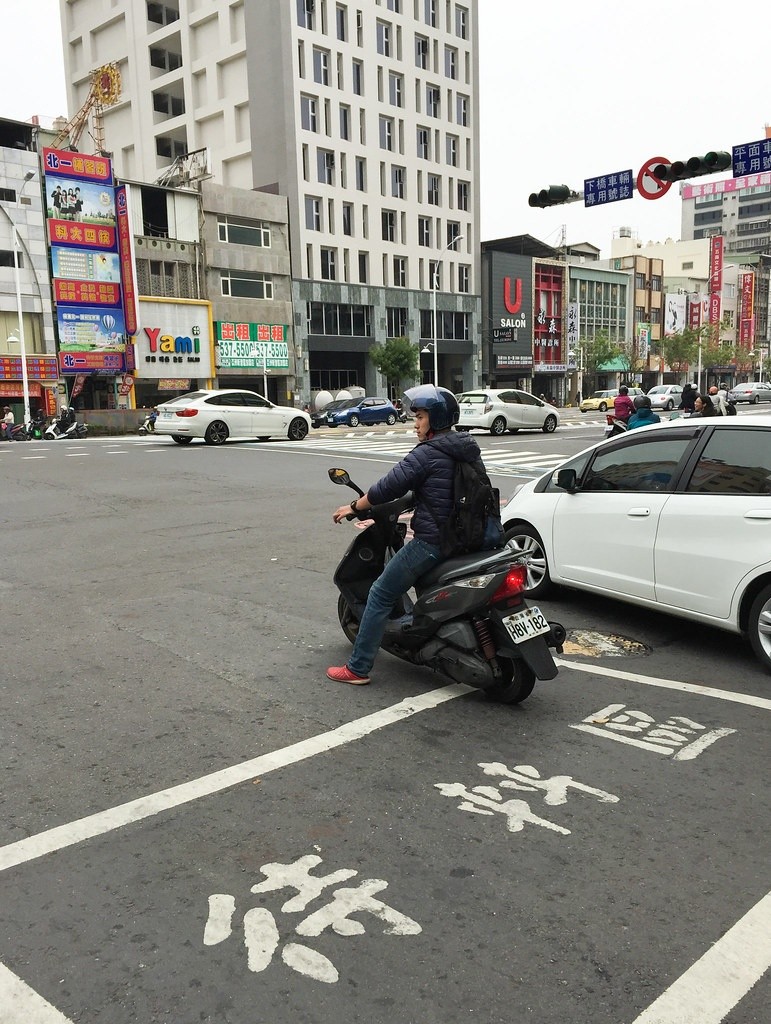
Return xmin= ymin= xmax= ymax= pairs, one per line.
xmin=654 ymin=151 xmax=731 ymax=183
xmin=527 ymin=185 xmax=569 ymax=209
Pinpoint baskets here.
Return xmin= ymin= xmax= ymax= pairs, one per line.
xmin=137 ymin=418 xmax=146 ymax=425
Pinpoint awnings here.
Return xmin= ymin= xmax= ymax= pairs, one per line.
xmin=593 ymin=354 xmax=631 ymax=373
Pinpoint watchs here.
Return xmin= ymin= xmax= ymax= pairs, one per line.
xmin=350 ymin=500 xmax=359 ymax=512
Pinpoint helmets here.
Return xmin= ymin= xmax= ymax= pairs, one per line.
xmin=69 ymin=407 xmax=74 ymax=411
xmin=410 ymin=386 xmax=460 ymax=431
xmin=691 ymin=383 xmax=698 ymax=390
xmin=685 ymin=383 xmax=691 ymax=390
xmin=619 ymin=385 xmax=629 ymax=395
xmin=710 ymin=386 xmax=717 ymax=394
xmin=633 ymin=396 xmax=651 ymax=409
xmin=61 ymin=405 xmax=67 ymax=410
xmin=721 ymin=382 xmax=728 ymax=388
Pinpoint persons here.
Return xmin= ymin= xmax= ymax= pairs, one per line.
xmin=68 ymin=407 xmax=76 ymax=422
xmin=681 ymin=383 xmax=701 ymax=408
xmin=0 ymin=406 xmax=14 ymax=442
xmin=695 ymin=396 xmax=717 ymax=416
xmin=149 ymin=406 xmax=158 ymax=430
xmin=628 ymin=396 xmax=660 ymax=430
xmin=718 ymin=383 xmax=738 ymax=415
xmin=575 ymin=391 xmax=580 ymax=407
xmin=614 ymin=385 xmax=635 ymax=423
xmin=708 ymin=386 xmax=728 ymax=416
xmin=35 ymin=409 xmax=46 ymax=440
xmin=328 ymin=384 xmax=491 ymax=684
xmin=57 ymin=405 xmax=71 ymax=435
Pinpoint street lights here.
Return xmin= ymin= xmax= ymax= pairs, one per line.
xmin=6 ymin=170 xmax=37 ymax=436
xmin=696 ymin=265 xmax=733 ymax=393
xmin=420 ymin=235 xmax=463 ymax=388
xmin=567 ymin=347 xmax=583 ymax=410
xmin=747 ymin=349 xmax=762 ymax=383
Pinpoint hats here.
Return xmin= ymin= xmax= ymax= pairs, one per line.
xmin=3 ymin=406 xmax=9 ymax=409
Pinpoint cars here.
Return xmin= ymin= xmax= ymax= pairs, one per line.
xmin=729 ymin=382 xmax=771 ymax=405
xmin=454 ymin=389 xmax=560 ymax=436
xmin=307 ymin=397 xmax=398 ymax=428
xmin=500 ymin=417 xmax=771 ymax=670
xmin=580 ymin=385 xmax=683 ymax=413
xmin=154 ymin=389 xmax=310 ymax=445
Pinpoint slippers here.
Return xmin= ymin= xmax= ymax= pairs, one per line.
xmin=327 ymin=664 xmax=371 ymax=685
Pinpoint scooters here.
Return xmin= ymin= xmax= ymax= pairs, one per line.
xmin=139 ymin=412 xmax=156 ymax=436
xmin=603 ymin=395 xmax=741 ymax=442
xmin=327 ymin=467 xmax=566 ymax=706
xmin=395 ymin=407 xmax=408 ymax=423
xmin=0 ymin=415 xmax=89 ymax=441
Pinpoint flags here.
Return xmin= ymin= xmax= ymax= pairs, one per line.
xmin=119 ymin=374 xmax=135 ymax=396
xmin=73 ymin=373 xmax=86 ymax=396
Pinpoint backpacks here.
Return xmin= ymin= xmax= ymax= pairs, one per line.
xmin=418 ymin=461 xmax=507 ymax=560
